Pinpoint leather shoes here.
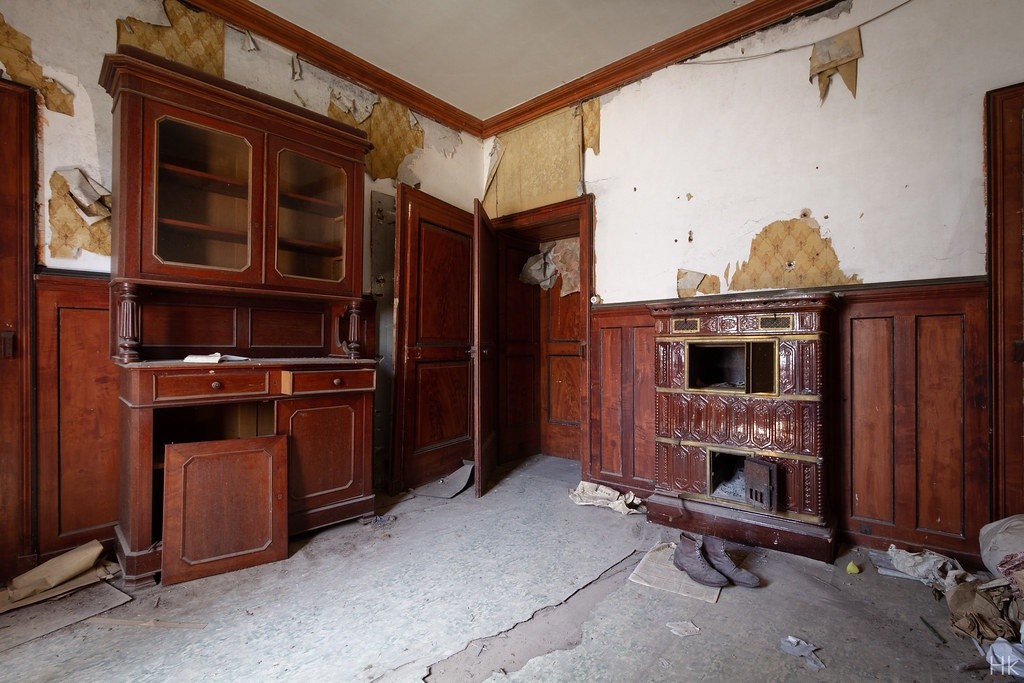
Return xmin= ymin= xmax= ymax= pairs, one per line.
xmin=703 ymin=534 xmax=761 ymax=587
xmin=673 ymin=532 xmax=729 ymax=587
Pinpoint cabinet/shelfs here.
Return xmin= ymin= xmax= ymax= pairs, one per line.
xmin=646 ymin=291 xmax=841 ymax=565
xmin=96 ymin=52 xmax=376 ymax=364
